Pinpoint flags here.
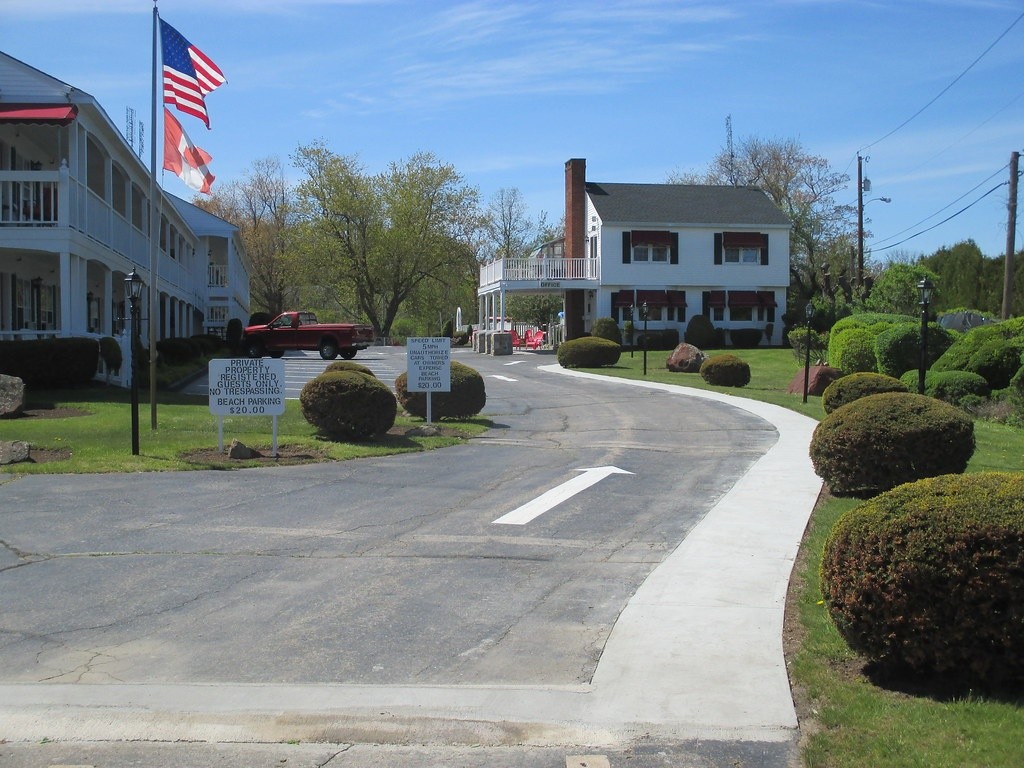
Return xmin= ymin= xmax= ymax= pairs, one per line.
xmin=160 ymin=19 xmax=228 ymax=130
xmin=163 ymin=108 xmax=215 ymax=196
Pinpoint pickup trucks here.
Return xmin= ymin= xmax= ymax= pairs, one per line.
xmin=237 ymin=309 xmax=374 ymax=360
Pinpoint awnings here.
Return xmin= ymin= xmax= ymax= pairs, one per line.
xmin=614 ymin=290 xmax=687 ymax=307
xmin=724 ymin=232 xmax=766 ymax=248
xmin=631 ymin=231 xmax=672 ymax=246
xmin=709 ymin=291 xmax=777 ymax=308
xmin=0 ymin=106 xmax=79 ymax=128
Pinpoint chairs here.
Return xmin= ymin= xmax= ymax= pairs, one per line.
xmin=509 ymin=329 xmax=522 ymax=351
xmin=525 ymin=329 xmax=544 ymax=350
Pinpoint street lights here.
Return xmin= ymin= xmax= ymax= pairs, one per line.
xmin=116 ymin=264 xmax=150 ymax=455
xmin=916 ymin=275 xmax=936 ymax=395
xmin=802 ymin=300 xmax=816 ymax=403
xmin=642 ymin=299 xmax=648 ymax=374
xmin=857 ymin=196 xmax=891 ymax=303
xmin=629 ymin=302 xmax=634 ymax=358
